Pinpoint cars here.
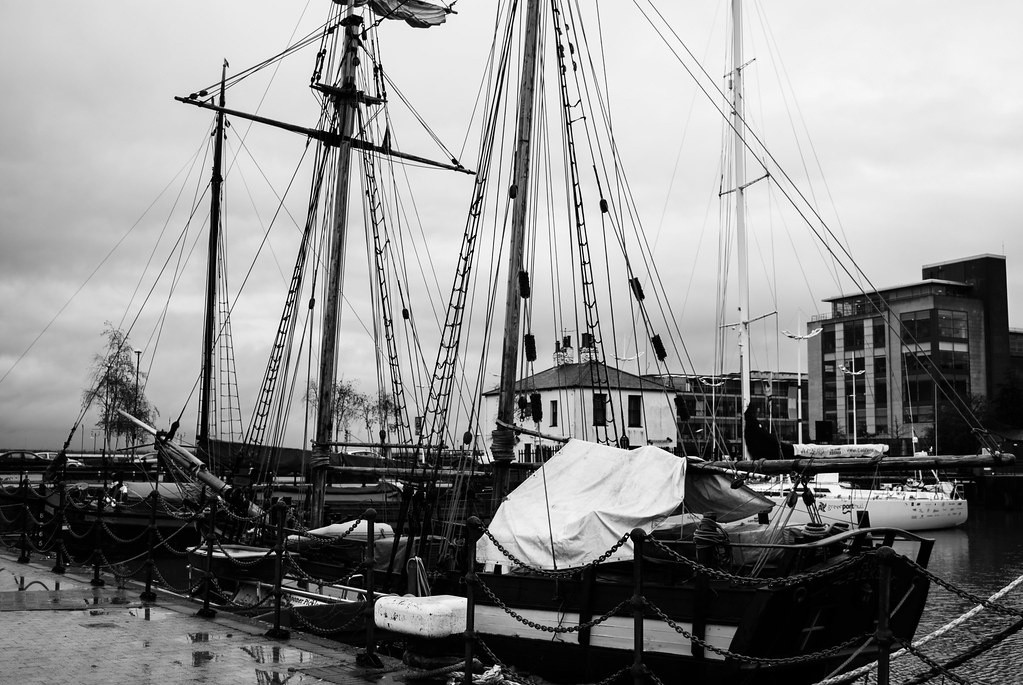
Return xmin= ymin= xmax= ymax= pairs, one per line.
xmin=0 ymin=451 xmax=84 ymax=474
xmin=126 ymin=452 xmax=157 ymax=467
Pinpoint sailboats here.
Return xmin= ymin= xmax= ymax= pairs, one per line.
xmin=0 ymin=0 xmax=1015 ymax=684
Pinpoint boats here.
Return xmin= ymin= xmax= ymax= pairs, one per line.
xmin=713 ymin=473 xmax=968 ymax=532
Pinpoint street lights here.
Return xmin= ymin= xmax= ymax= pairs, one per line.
xmin=839 ymin=364 xmax=866 ymax=445
xmin=781 ymin=328 xmax=822 ymax=443
